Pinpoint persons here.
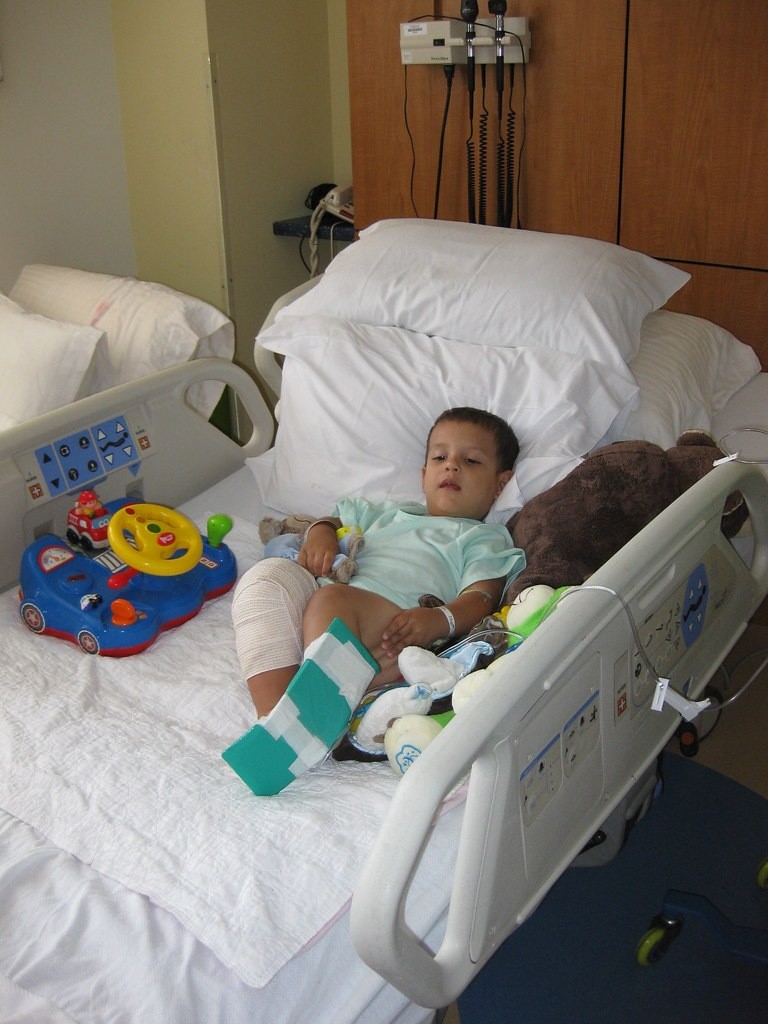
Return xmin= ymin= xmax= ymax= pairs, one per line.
xmin=231 ymin=407 xmax=527 ymax=723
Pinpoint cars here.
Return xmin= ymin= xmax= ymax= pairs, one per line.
xmin=66 ymin=495 xmax=114 ymax=551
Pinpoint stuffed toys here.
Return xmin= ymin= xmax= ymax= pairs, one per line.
xmin=332 ymin=585 xmax=571 ymax=781
xmin=503 ymin=428 xmax=751 ymax=610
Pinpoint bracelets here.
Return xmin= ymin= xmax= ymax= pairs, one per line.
xmin=303 ymin=519 xmax=339 ymax=543
xmin=433 ymin=605 xmax=455 ymax=641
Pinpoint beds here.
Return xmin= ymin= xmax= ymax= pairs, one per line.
xmin=0 ymin=217 xmax=768 ymax=1024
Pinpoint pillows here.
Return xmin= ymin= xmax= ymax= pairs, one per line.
xmin=8 ymin=264 xmax=235 ymax=421
xmin=246 ymin=317 xmax=640 ymax=526
xmin=587 ymin=308 xmax=761 ymax=462
xmin=274 ymin=218 xmax=692 ymax=385
xmin=0 ymin=290 xmax=108 ymax=430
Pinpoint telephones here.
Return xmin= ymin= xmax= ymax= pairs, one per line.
xmin=320 ymin=168 xmax=354 ymax=226
